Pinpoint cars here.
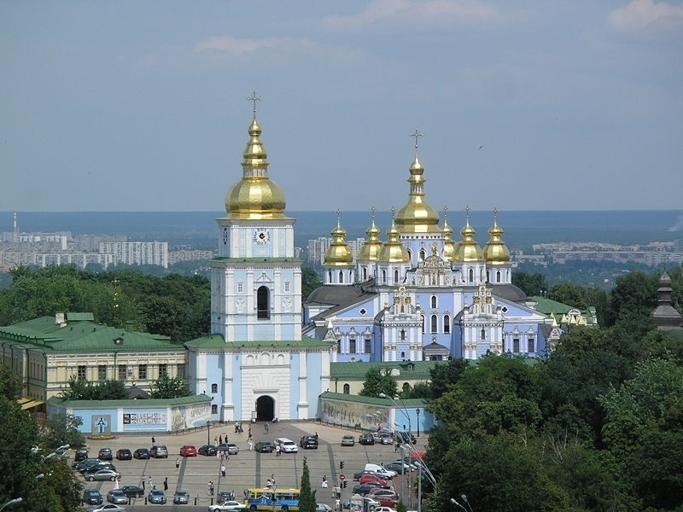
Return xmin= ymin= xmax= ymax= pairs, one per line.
xmin=71 ymin=444 xmax=129 ymax=512
xmin=132 ymin=432 xmax=319 ymax=460
xmin=341 ymin=428 xmax=418 ymax=448
xmin=119 ymin=485 xmax=248 ymax=511
xmin=314 ymin=451 xmax=425 ymax=512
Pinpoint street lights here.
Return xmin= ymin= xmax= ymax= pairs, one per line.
xmin=0 ymin=496 xmax=23 ymax=511
xmin=397 ymin=442 xmax=473 ymax=512
xmin=206 ymin=419 xmax=211 ymax=445
xmin=366 ymin=392 xmax=412 ymax=427
xmin=30 ymin=441 xmax=71 ymax=479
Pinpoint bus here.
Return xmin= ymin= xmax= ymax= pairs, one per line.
xmin=240 ymin=487 xmax=301 ymax=512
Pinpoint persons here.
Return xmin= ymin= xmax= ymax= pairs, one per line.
xmin=321 ymin=473 xmax=327 ymax=488
xmin=162 ymin=477 xmax=168 ymax=490
xmin=334 ymin=496 xmax=340 ymax=511
xmin=174 ymin=457 xmax=179 ymax=469
xmin=265 ymin=478 xmax=275 ymax=489
xmin=220 ymin=464 xmax=224 ymax=476
xmin=207 ymin=480 xmax=215 ymax=496
xmin=335 ymin=484 xmax=340 ymax=499
xmin=212 ymin=417 xmax=284 ymax=463
xmin=330 ymin=484 xmax=336 ymax=497
xmin=145 ymin=475 xmax=153 ymax=489
xmin=151 ymin=435 xmax=155 ymax=446
